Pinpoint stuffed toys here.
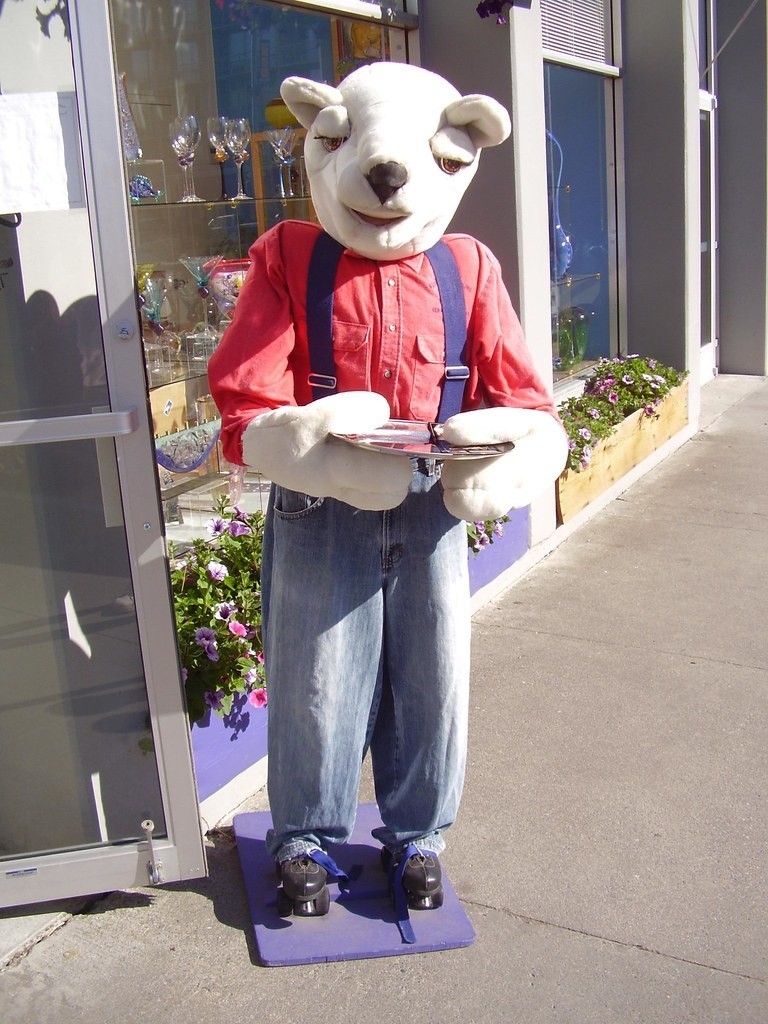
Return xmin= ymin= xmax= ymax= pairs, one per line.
xmin=206 ymin=62 xmax=570 ymax=901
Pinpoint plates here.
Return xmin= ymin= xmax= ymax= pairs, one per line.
xmin=330 ymin=420 xmax=515 ymax=460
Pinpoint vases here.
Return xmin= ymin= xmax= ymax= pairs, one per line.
xmin=554 ymin=376 xmax=688 ymax=524
xmin=191 ymin=495 xmax=531 ymax=837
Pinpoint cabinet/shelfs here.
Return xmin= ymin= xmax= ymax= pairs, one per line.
xmin=250 ymin=127 xmax=316 ymax=239
xmin=124 ymin=196 xmax=312 ymax=559
xmin=549 ymin=183 xmax=602 ymax=385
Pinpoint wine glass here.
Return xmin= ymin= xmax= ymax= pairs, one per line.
xmin=169 ymin=115 xmax=299 ymax=203
xmin=136 ymin=255 xmax=225 ymax=351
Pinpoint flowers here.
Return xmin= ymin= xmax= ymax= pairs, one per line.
xmin=167 ymin=494 xmax=511 ymax=733
xmin=556 ymin=349 xmax=690 ymax=475
